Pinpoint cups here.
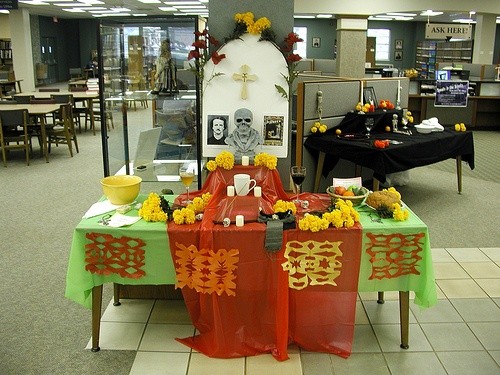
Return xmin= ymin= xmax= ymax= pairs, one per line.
xmin=234 ymin=173 xmax=256 ymax=196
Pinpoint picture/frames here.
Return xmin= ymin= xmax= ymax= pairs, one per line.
xmin=394 ymin=40 xmax=403 ymax=61
xmin=312 ymin=37 xmax=321 ymax=48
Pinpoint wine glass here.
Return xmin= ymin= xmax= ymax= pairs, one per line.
xmin=291 ymin=166 xmax=307 ymax=203
xmin=402 ymin=109 xmax=409 ymax=129
xmin=179 ymin=167 xmax=195 ymax=203
xmin=365 ymin=118 xmax=374 ymax=137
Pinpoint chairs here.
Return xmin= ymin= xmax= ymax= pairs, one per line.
xmin=0 ymin=63 xmax=148 ymax=167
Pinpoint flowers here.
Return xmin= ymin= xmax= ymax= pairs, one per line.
xmin=335 ymin=129 xmax=342 ymax=135
xmin=311 ymin=122 xmax=327 ymax=134
xmin=373 ymin=140 xmax=390 ymax=149
xmin=137 ymin=151 xmax=409 ymax=232
xmin=355 ymin=101 xmax=375 ymax=114
xmin=379 ymin=99 xmax=395 ymax=110
xmin=404 ymin=110 xmax=413 ymax=123
xmin=455 ymin=122 xmax=467 ymax=132
xmin=187 ymin=11 xmax=304 ymax=104
xmin=385 ymin=126 xmax=390 ymax=132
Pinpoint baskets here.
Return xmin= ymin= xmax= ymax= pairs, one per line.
xmin=325 ymin=186 xmax=369 ymax=207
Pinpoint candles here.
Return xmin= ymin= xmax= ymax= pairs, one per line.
xmin=361 ymin=82 xmax=363 ymax=105
xmin=398 ymin=80 xmax=400 ymax=102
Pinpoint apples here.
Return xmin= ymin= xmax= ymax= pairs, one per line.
xmin=335 ymin=185 xmax=359 ymax=196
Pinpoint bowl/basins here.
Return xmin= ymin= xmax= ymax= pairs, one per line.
xmin=100 ymin=175 xmax=142 ymax=205
xmin=326 ymin=186 xmax=368 ymax=206
xmin=414 ymin=125 xmax=435 ymax=133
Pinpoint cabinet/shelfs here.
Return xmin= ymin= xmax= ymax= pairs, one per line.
xmin=96 ymin=15 xmax=207 ymax=190
xmin=415 ymin=41 xmax=474 ymax=79
xmin=0 ymin=38 xmax=13 ymax=69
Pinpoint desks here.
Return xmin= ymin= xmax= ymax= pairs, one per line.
xmin=1 ymin=92 xmax=100 ymax=135
xmin=0 ymin=104 xmax=66 ymax=163
xmin=0 ymin=79 xmax=23 ymax=93
xmin=64 ymin=193 xmax=437 ymax=350
xmin=83 ymin=66 xmax=121 ymax=79
xmin=291 ymin=60 xmax=500 ymax=194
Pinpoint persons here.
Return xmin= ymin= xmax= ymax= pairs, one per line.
xmin=209 ymin=117 xmax=228 ymax=145
xmin=368 ymin=95 xmax=374 ymax=105
